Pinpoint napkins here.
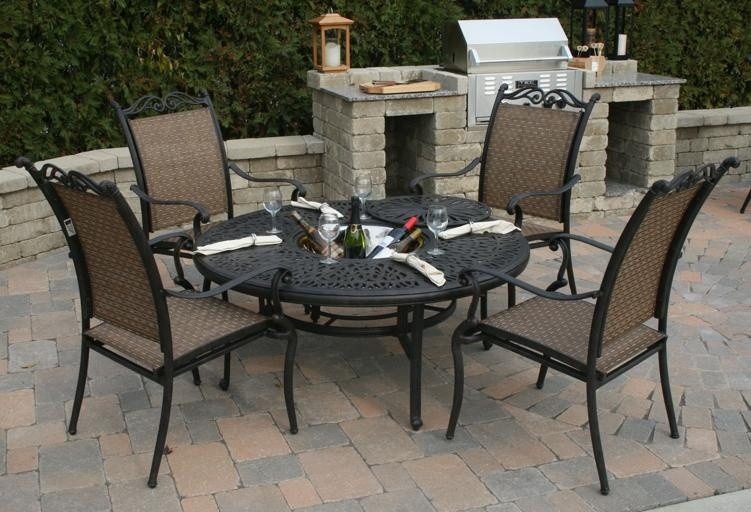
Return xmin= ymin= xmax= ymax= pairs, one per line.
xmin=291 ymin=197 xmax=344 ymax=218
xmin=190 ymin=233 xmax=282 ymax=256
xmin=391 ymin=251 xmax=446 ymax=288
xmin=438 ymin=220 xmax=522 ymax=240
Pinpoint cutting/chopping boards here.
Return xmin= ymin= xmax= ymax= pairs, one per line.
xmin=358 ymin=78 xmax=441 ymax=93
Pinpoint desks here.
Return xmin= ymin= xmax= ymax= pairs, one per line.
xmin=195 ymin=194 xmax=530 ymax=434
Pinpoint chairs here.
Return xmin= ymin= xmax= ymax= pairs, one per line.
xmin=16 ymin=156 xmax=297 ymax=489
xmin=409 ymin=84 xmax=601 ymax=348
xmin=109 ymin=86 xmax=306 ymax=330
xmin=445 ymin=157 xmax=743 ymax=493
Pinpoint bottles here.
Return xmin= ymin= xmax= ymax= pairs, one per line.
xmin=291 ymin=196 xmax=423 ymax=261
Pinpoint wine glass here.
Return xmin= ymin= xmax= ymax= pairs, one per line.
xmin=426 ymin=205 xmax=446 ymax=256
xmin=354 ymin=173 xmax=372 ymax=219
xmin=318 ymin=211 xmax=340 ymax=265
xmin=263 ymin=184 xmax=284 ymax=235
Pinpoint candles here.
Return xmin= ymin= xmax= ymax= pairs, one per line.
xmin=617 ymin=33 xmax=628 ymax=55
xmin=325 ymin=42 xmax=341 ymax=67
